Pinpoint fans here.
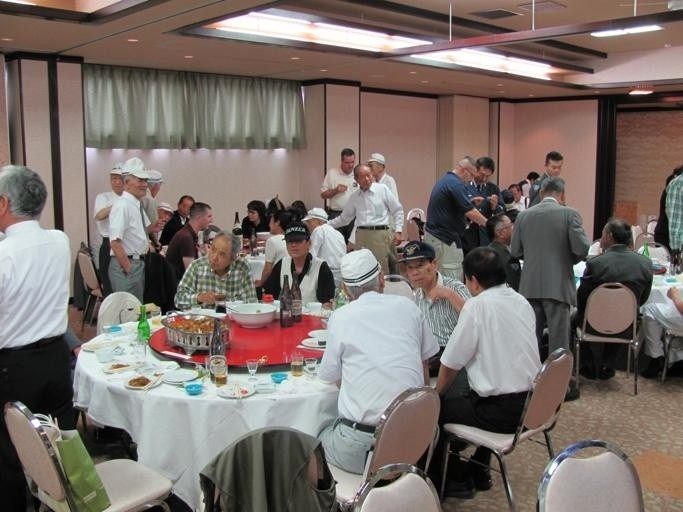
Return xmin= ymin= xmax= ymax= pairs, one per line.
xmin=616 ymin=0 xmax=683 ymax=11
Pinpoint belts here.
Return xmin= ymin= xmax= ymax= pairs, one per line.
xmin=358 ymin=225 xmax=389 ymax=230
xmin=337 ymin=418 xmax=375 ymax=433
xmin=128 ymin=255 xmax=144 ymax=259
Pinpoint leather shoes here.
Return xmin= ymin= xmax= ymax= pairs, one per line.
xmin=641 ymin=356 xmax=683 ymax=378
xmin=445 ymin=468 xmax=492 ymax=499
xmin=565 ymin=388 xmax=579 ymax=402
xmin=579 ymin=364 xmax=615 ymax=379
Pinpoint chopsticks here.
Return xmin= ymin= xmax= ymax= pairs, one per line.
xmin=233 ymin=384 xmax=242 ymax=398
xmin=142 ymin=374 xmax=161 ymax=391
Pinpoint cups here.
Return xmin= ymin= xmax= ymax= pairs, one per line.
xmin=102 ymin=322 xmax=112 ymax=340
xmin=150 ymin=307 xmax=161 ymax=329
xmin=289 ymin=353 xmax=304 ymax=379
xmin=306 ymin=302 xmax=322 ymax=312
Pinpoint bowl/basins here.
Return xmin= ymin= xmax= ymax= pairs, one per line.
xmin=269 ymin=372 xmax=288 ymax=381
xmin=255 ymin=232 xmax=269 ymax=242
xmin=95 ymin=348 xmax=113 ymax=363
xmin=183 ymin=383 xmax=204 ymax=396
xmin=227 ymin=303 xmax=276 ymax=328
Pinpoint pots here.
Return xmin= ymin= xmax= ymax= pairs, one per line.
xmin=160 ymin=310 xmax=231 ymax=335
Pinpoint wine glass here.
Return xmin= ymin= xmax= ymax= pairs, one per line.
xmin=305 ymin=358 xmax=317 ymax=383
xmin=246 ymin=359 xmax=258 ymax=382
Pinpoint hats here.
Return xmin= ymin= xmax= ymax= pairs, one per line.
xmin=301 ymin=207 xmax=329 ymax=223
xmin=281 ymin=223 xmax=310 ymax=240
xmin=158 ymin=202 xmax=174 ymax=214
xmin=396 ymin=241 xmax=435 ymax=263
xmin=110 ymin=157 xmax=162 ymax=183
xmin=367 ymin=154 xmax=385 ymax=165
xmin=341 ymin=249 xmax=381 ymax=287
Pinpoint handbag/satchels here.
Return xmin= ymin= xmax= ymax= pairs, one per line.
xmin=33 ymin=413 xmax=111 ymax=512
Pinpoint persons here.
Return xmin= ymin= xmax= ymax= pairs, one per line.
xmin=241 ymin=200 xmax=347 ymax=310
xmin=315 ymin=248 xmax=441 ymax=475
xmin=321 ymin=148 xmax=403 ymax=281
xmin=577 ymin=218 xmax=653 ymax=380
xmin=64 ymin=326 xmax=83 ymax=430
xmin=140 ymin=169 xmax=165 ymax=253
xmin=0 ymin=165 xmax=73 ymax=512
xmin=158 ymin=195 xmax=214 ymax=281
xmin=428 ymin=246 xmax=543 ymax=499
xmin=654 ymin=164 xmax=683 ymax=252
xmin=641 ymin=284 xmax=683 ymax=379
xmin=92 ymin=161 xmax=124 ymax=301
xmin=510 ymin=176 xmax=590 ymax=403
xmin=108 ymin=157 xmax=167 ymax=306
xmin=394 ymin=240 xmax=472 ymax=378
xmin=422 ymin=151 xmax=564 ymax=292
xmin=174 ymin=232 xmax=258 ymax=311
xmin=663 ymin=171 xmax=683 ymax=273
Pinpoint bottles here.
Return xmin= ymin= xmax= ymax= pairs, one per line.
xmin=291 ymin=273 xmax=302 ymax=321
xmin=231 ymin=211 xmax=243 ymax=251
xmin=279 ymin=274 xmax=293 ymax=327
xmin=640 ymin=239 xmax=650 ymax=257
xmin=249 ymin=228 xmax=257 ymax=258
xmin=136 ymin=306 xmax=150 ymax=344
xmin=209 ymin=318 xmax=226 ymax=385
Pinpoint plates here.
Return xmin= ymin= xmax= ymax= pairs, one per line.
xmin=219 ymin=382 xmax=256 ymax=398
xmin=300 ymin=329 xmax=328 ymax=348
xmin=81 ymin=332 xmax=199 ymax=391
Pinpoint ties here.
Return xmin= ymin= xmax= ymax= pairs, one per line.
xmin=477 ymin=185 xmax=480 ymax=192
